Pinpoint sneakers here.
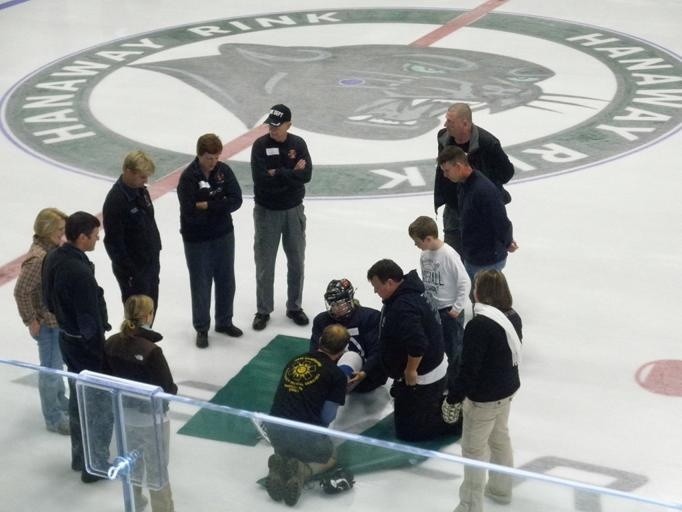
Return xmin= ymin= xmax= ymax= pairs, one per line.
xmin=485 ymin=484 xmax=512 ymax=504
xmin=283 ymin=457 xmax=307 ymax=507
xmin=45 ymin=414 xmax=73 ymax=435
xmin=81 ymin=463 xmax=117 ymax=483
xmin=124 ymin=495 xmax=148 ymax=511
xmin=265 ymin=454 xmax=284 ymax=501
xmin=72 ymin=459 xmax=85 ymax=471
xmin=61 ymin=399 xmax=71 ymax=412
xmin=451 ymin=501 xmax=484 ymax=512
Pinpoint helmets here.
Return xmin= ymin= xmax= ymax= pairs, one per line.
xmin=324 ymin=278 xmax=356 ymax=321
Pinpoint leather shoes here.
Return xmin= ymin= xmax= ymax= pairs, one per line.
xmin=286 ymin=307 xmax=309 ymax=326
xmin=215 ymin=323 xmax=243 ymax=337
xmin=196 ymin=330 xmax=208 ymax=347
xmin=253 ymin=312 xmax=270 ymax=330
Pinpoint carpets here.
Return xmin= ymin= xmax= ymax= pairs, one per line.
xmin=256 ymin=412 xmax=460 ymax=495
xmin=176 ymin=334 xmax=310 ymax=446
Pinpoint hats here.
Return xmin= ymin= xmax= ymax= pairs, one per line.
xmin=263 ymin=104 xmax=291 ymax=127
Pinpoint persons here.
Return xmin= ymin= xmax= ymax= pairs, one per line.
xmin=440 ymin=268 xmax=523 ymax=511
xmin=176 ymin=133 xmax=243 ymax=348
xmin=102 ymin=149 xmax=162 ymax=327
xmin=12 ymin=207 xmax=70 ymax=435
xmin=433 ymin=102 xmax=515 ymax=262
xmin=408 ymin=215 xmax=473 ymax=372
xmin=309 ymin=278 xmax=390 ymax=394
xmin=250 ymin=103 xmax=313 ymax=330
xmin=436 ymin=144 xmax=519 ymax=306
xmin=366 ymin=258 xmax=463 ymax=442
xmin=103 ymin=293 xmax=178 ymax=512
xmin=40 ymin=210 xmax=116 ymax=484
xmin=263 ymin=323 xmax=352 ymax=507
